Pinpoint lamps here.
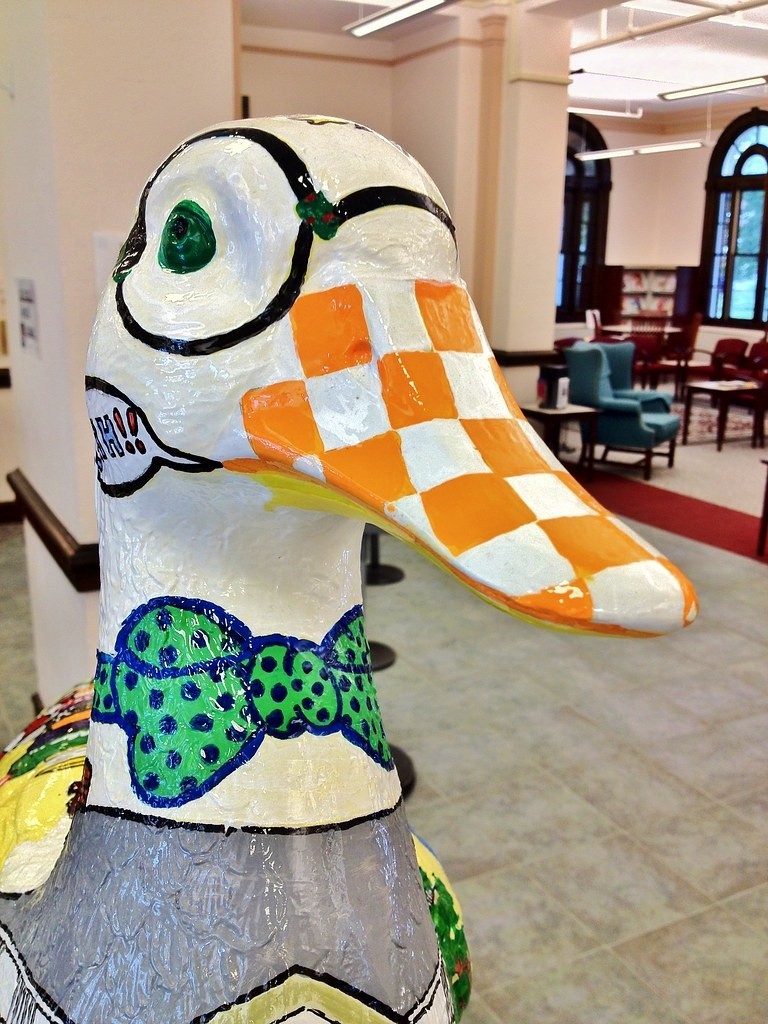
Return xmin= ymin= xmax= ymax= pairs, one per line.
xmin=342 ymin=0 xmax=461 ymax=40
xmin=568 ymin=98 xmax=643 ymax=118
xmin=575 ymin=96 xmax=716 ymax=162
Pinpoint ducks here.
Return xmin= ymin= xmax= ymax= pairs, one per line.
xmin=0 ymin=111 xmax=698 ymax=1023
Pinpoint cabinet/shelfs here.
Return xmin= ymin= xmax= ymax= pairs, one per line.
xmin=581 ymin=264 xmax=705 ymax=321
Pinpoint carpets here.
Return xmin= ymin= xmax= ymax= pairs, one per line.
xmin=659 ymin=403 xmax=768 ymax=447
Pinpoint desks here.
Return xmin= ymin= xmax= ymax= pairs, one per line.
xmin=518 ymin=402 xmax=601 ymax=462
xmin=682 ymin=381 xmax=768 ymax=452
xmin=602 ymin=325 xmax=682 ymax=356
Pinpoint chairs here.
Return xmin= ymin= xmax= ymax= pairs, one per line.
xmin=584 ymin=310 xmax=601 ymax=341
xmin=633 ymin=313 xmax=702 ymax=389
xmin=562 ymin=341 xmax=681 ymax=481
xmin=684 ymin=339 xmax=749 ymax=380
